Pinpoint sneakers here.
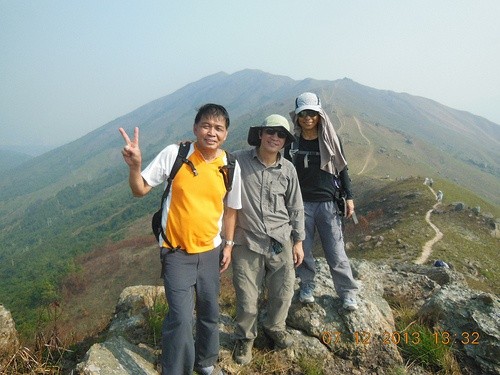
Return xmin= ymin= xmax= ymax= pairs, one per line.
xmin=299 ymin=288 xmax=314 ymax=305
xmin=341 ymin=296 xmax=358 ymax=310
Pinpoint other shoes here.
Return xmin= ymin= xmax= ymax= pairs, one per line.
xmin=234 ymin=338 xmax=254 ymax=365
xmin=200 ymin=365 xmax=223 ymax=375
xmin=263 ymin=326 xmax=295 ymax=349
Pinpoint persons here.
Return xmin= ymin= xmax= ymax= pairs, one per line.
xmin=282 ymin=91 xmax=360 ymax=312
xmin=224 ymin=113 xmax=307 ymax=365
xmin=117 ymin=103 xmax=244 ymax=375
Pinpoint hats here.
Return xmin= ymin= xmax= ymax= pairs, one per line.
xmin=294 ymin=92 xmax=322 ymax=114
xmin=248 ymin=114 xmax=296 ymax=149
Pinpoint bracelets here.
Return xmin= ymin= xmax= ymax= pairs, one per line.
xmin=224 ymin=239 xmax=235 ymax=246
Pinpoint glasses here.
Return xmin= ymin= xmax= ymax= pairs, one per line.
xmin=298 ymin=110 xmax=317 ymax=118
xmin=265 ymin=128 xmax=287 ymax=138
